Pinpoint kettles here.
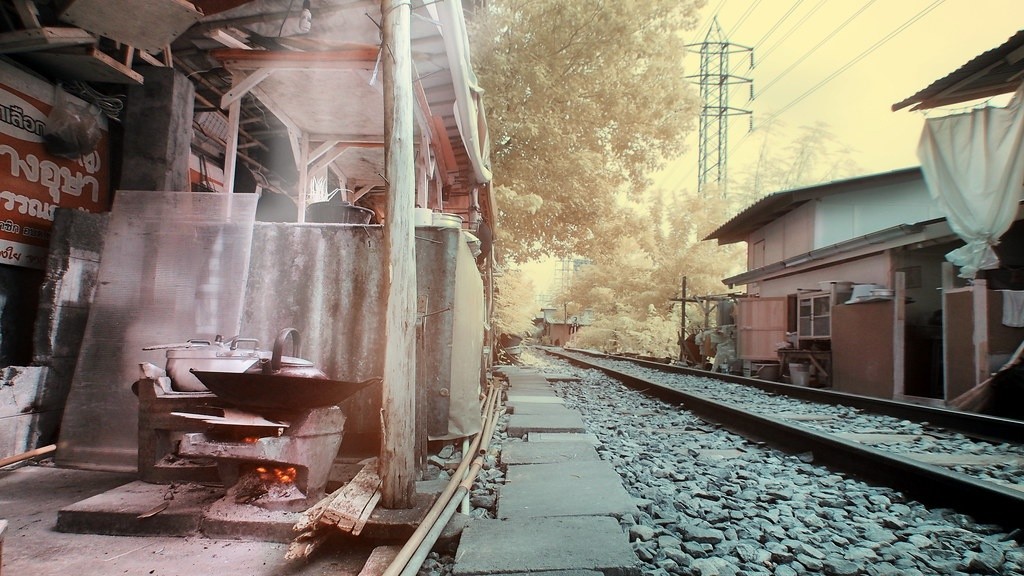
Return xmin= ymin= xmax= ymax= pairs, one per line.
xmin=241 ymin=327 xmax=333 ymax=380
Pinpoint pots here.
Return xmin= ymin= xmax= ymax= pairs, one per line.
xmin=165 ymin=334 xmax=273 ymax=391
xmin=432 ymin=207 xmax=465 ymax=229
xmin=189 ymin=368 xmax=384 ymax=416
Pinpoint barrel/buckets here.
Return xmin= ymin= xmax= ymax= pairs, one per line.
xmin=788 ymin=363 xmax=811 ymax=388
xmin=755 ymin=364 xmax=780 ymax=381
